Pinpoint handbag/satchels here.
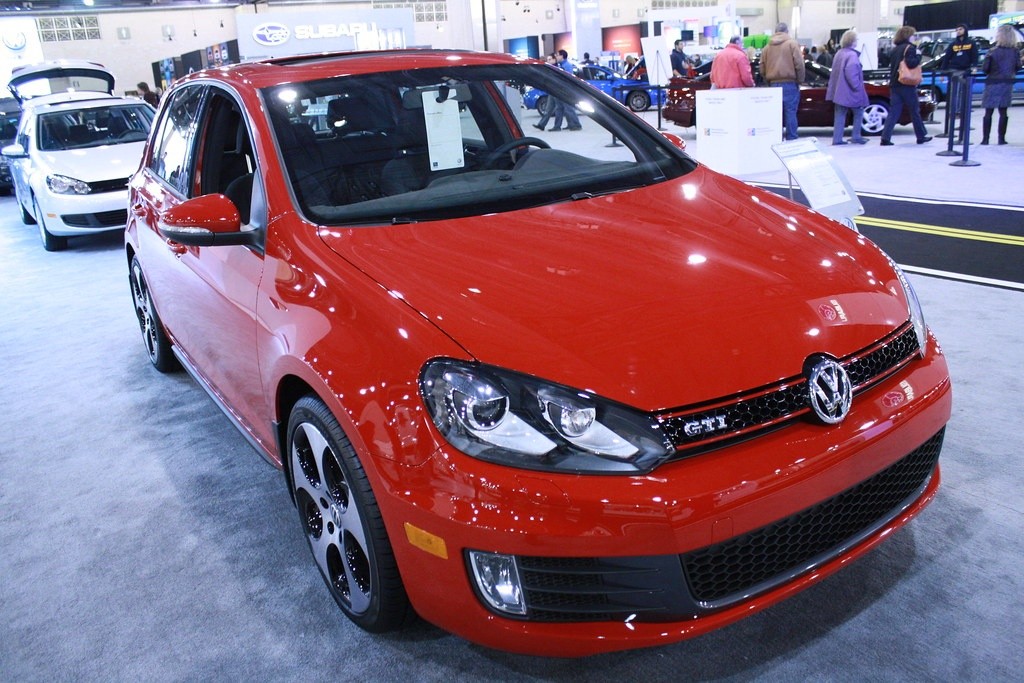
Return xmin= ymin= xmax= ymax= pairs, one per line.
xmin=898 ymin=44 xmax=922 ymax=85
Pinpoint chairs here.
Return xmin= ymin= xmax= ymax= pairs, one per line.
xmin=104 ymin=116 xmax=130 ymax=138
xmin=382 ymin=108 xmax=474 ymax=193
xmin=223 ymin=102 xmax=331 ymax=214
xmin=43 ymin=121 xmax=70 ymax=145
xmin=67 ymin=125 xmax=90 ymax=137
xmin=94 ymin=112 xmax=114 ymax=135
xmin=329 ymin=95 xmax=387 ymax=162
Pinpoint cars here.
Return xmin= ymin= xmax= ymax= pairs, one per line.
xmin=921 ymin=48 xmax=1024 ymax=106
xmin=121 ymin=48 xmax=955 ymax=660
xmin=522 ymin=62 xmax=667 ymax=117
xmin=662 ymin=52 xmax=938 ymax=138
xmin=0 ymin=60 xmax=159 ymax=252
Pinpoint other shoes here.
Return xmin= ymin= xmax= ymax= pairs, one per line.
xmin=548 ymin=127 xmax=561 ymax=131
xmin=917 ymin=136 xmax=932 ymax=144
xmin=563 ymin=126 xmax=569 ymax=129
xmin=880 ymin=139 xmax=894 ymax=145
xmin=836 ymin=139 xmax=865 ymax=144
xmin=570 ymin=126 xmax=582 ymax=130
xmin=533 ymin=124 xmax=544 ymax=130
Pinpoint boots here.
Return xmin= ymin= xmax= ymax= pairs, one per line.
xmin=980 ymin=117 xmax=992 ymax=145
xmin=998 ymin=117 xmax=1009 ymax=144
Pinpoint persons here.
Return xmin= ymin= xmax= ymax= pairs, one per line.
xmin=880 ymin=26 xmax=933 ymax=146
xmin=623 ymin=55 xmax=638 ymax=79
xmin=532 ymin=49 xmax=601 ymax=132
xmin=759 ymin=21 xmax=806 ymax=140
xmin=825 ymin=30 xmax=870 ymax=144
xmin=981 ymin=25 xmax=1023 ymax=146
xmin=670 ymin=39 xmax=703 ymax=78
xmin=800 ymin=38 xmax=841 ymax=69
xmin=710 ymin=35 xmax=756 ymax=89
xmin=135 ymin=82 xmax=162 ymax=109
xmin=939 ymin=24 xmax=979 ymax=116
